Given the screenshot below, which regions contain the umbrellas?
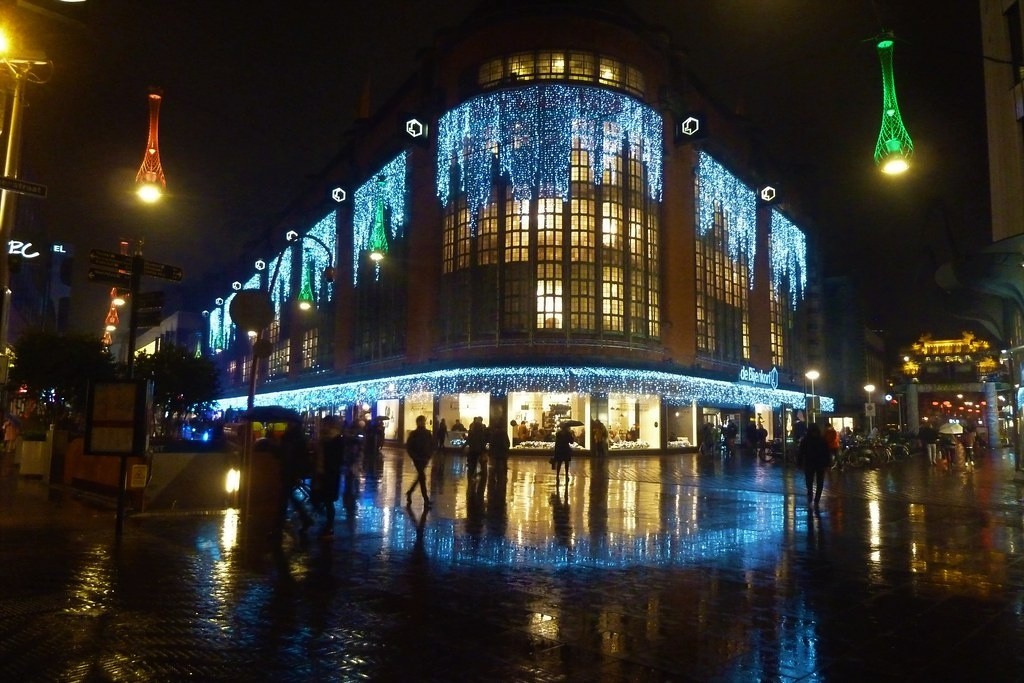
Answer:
[556,419,584,428]
[6,413,21,426]
[374,415,390,421]
[939,423,964,434]
[236,405,304,438]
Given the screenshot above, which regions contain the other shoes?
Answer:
[320,528,334,536]
[965,462,969,467]
[555,477,560,481]
[930,460,937,465]
[298,518,315,533]
[807,495,820,505]
[406,491,414,504]
[971,461,974,465]
[424,501,435,507]
[566,477,570,481]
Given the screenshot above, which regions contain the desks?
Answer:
[447,431,467,440]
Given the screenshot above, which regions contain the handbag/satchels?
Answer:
[549,459,557,470]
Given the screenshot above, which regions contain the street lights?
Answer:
[0,27,51,288]
[885,393,894,402]
[932,393,988,412]
[805,371,820,423]
[121,181,167,369]
[104,297,127,332]
[864,385,875,433]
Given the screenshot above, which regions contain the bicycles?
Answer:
[759,435,912,466]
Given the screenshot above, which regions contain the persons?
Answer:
[555,424,574,485]
[405,416,433,507]
[944,433,955,467]
[176,402,885,473]
[310,420,345,535]
[257,420,316,540]
[797,423,829,503]
[961,427,975,466]
[925,421,937,465]
[0,415,20,451]
